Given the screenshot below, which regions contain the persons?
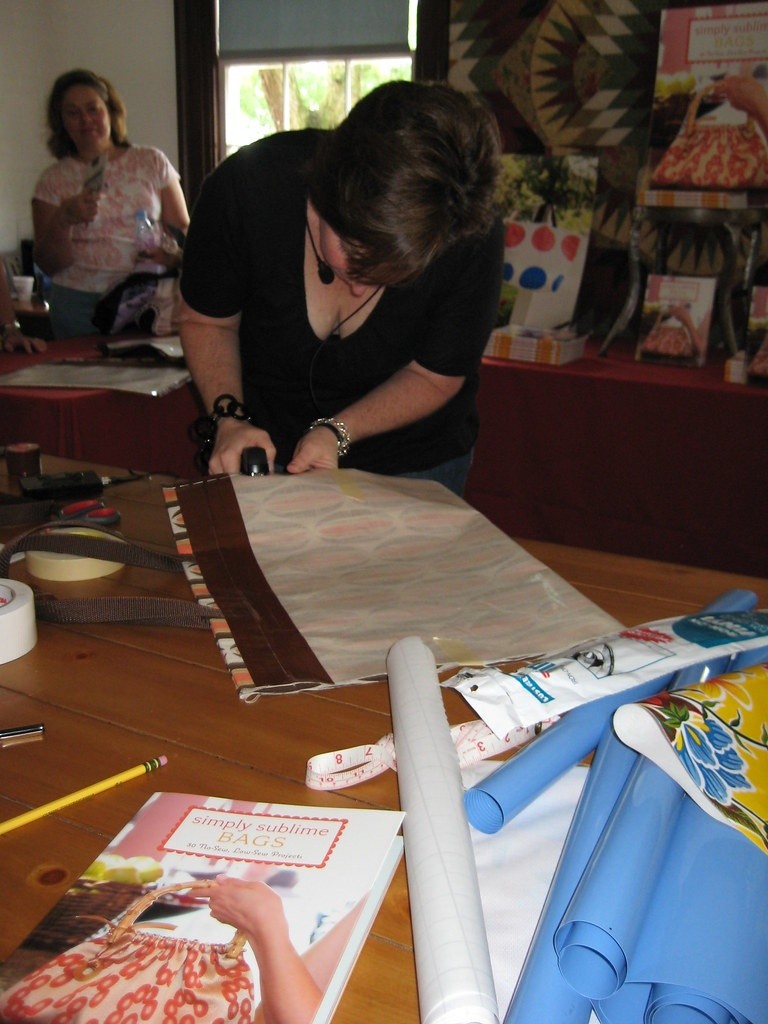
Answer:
[189,875,368,1024]
[32,70,191,336]
[726,76,768,124]
[0,260,47,355]
[182,80,502,498]
[665,307,707,354]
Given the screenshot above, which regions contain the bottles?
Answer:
[135,209,157,276]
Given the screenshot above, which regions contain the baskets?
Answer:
[23,877,157,950]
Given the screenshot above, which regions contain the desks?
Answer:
[0,341,768,579]
[0,453,768,1024]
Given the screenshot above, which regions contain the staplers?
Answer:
[241,446,269,477]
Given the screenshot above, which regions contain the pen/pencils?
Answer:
[0,723,45,740]
[63,357,123,365]
[0,754,168,835]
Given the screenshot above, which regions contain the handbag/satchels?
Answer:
[642,310,691,357]
[502,199,590,333]
[654,79,768,189]
[91,226,187,335]
[0,879,255,1024]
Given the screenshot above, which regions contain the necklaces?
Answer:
[330,283,383,333]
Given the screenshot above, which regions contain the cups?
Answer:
[12,276,34,303]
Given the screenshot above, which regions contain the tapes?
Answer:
[0,578,38,664]
[25,527,128,582]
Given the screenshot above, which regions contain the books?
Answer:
[645,5,768,186]
[745,286,768,377]
[0,792,406,1024]
[636,274,717,367]
[639,189,748,208]
[484,325,588,365]
[723,350,748,385]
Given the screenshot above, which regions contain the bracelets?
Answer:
[306,418,351,457]
[3,322,20,333]
[195,394,252,442]
